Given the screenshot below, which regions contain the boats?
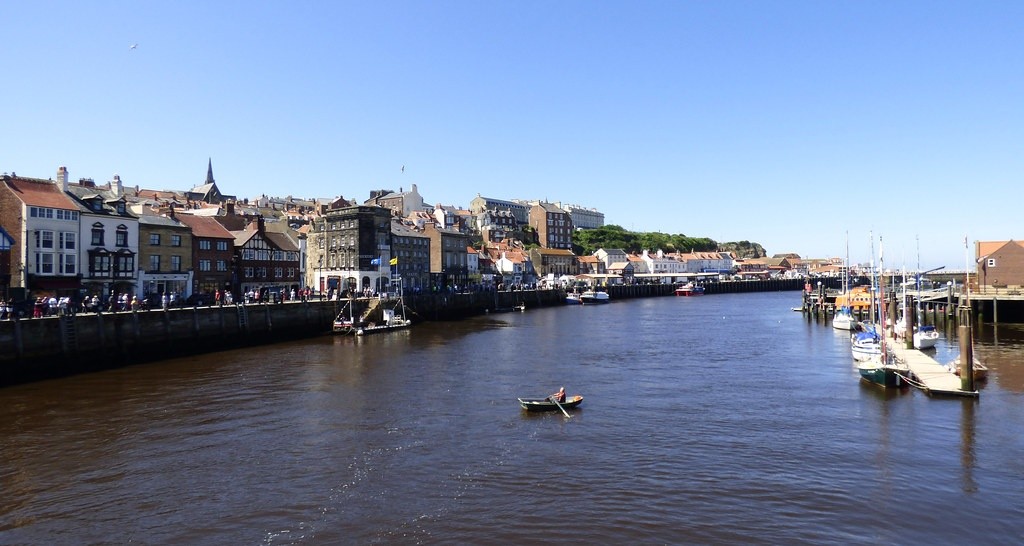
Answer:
[675,282,705,297]
[333,289,365,333]
[833,227,989,383]
[566,289,582,304]
[355,295,411,336]
[517,395,584,412]
[580,286,609,305]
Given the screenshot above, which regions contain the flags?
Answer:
[389,258,397,265]
[371,259,380,265]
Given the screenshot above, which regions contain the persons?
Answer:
[0,293,148,321]
[282,286,374,302]
[554,387,566,403]
[377,282,546,299]
[214,289,221,306]
[225,290,268,305]
[162,292,168,308]
[169,291,178,309]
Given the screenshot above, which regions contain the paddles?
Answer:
[552,396,570,419]
